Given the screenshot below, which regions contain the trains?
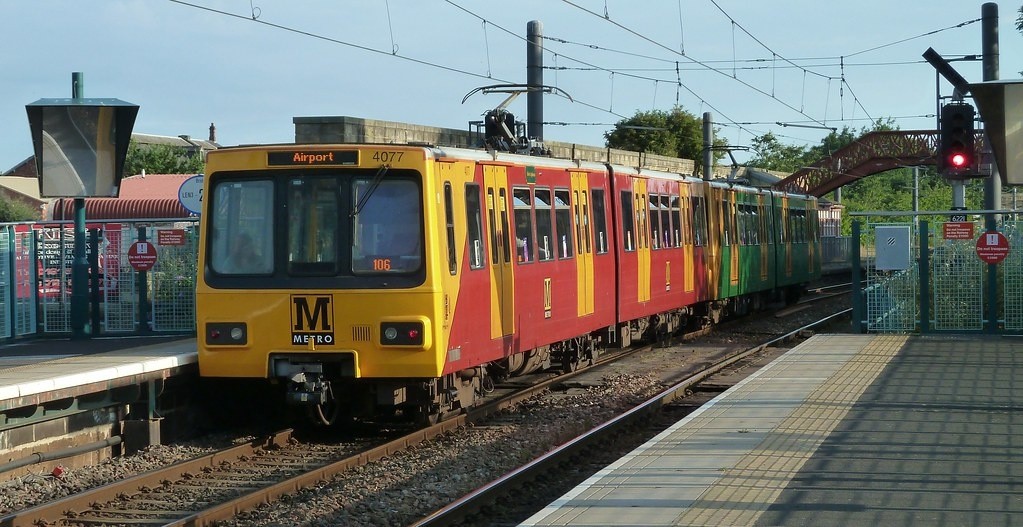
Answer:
[195,141,823,428]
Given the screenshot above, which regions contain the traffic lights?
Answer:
[940,103,974,176]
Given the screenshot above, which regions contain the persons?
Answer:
[222,234,262,273]
